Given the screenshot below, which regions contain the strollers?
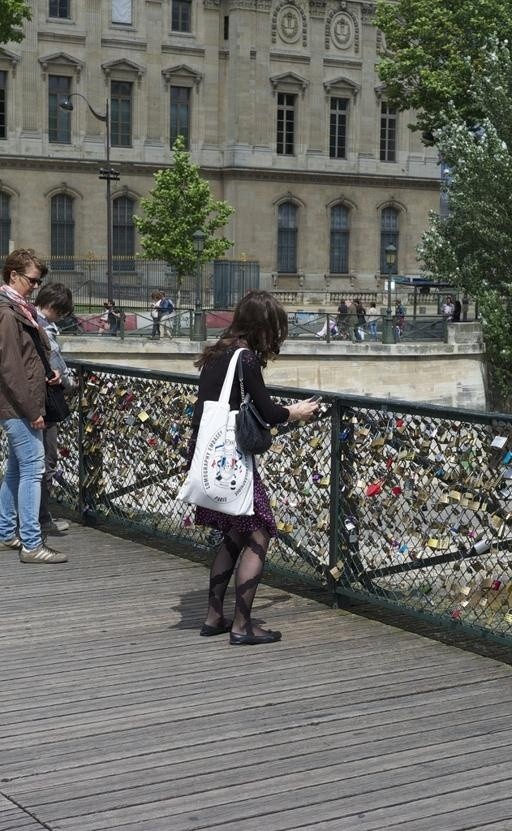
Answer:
[313,316,350,342]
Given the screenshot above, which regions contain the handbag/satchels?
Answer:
[46,372,69,422]
[175,401,255,516]
[236,392,271,455]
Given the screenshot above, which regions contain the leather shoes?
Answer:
[230,631,281,644]
[200,620,233,636]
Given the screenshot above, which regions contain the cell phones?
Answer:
[308,394,323,403]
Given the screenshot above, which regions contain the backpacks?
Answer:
[168,303,173,313]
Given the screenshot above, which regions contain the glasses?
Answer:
[18,272,42,286]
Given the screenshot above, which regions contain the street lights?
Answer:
[188,225,211,343]
[380,241,401,345]
[60,92,121,323]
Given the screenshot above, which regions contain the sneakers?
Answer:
[0,537,22,550]
[41,522,69,531]
[20,543,67,563]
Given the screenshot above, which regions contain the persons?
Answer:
[107,299,121,336]
[149,291,161,338]
[98,302,111,337]
[441,296,461,344]
[0,248,67,563]
[156,290,173,340]
[187,291,317,646]
[336,296,406,341]
[33,281,75,533]
